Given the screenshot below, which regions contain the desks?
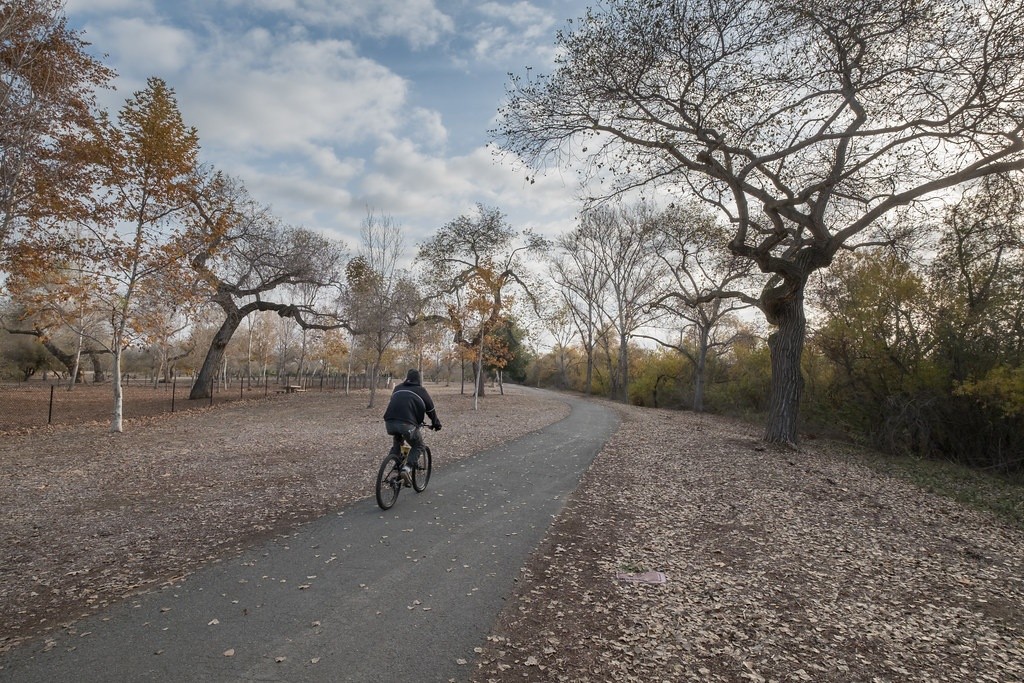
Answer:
[283,386,301,394]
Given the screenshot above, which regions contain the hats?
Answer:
[407,369,420,382]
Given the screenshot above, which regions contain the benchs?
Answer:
[273,391,285,392]
[295,390,307,392]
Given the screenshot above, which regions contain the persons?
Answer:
[383,369,442,473]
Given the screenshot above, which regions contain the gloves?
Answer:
[431,420,442,432]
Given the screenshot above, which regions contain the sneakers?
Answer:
[401,468,413,489]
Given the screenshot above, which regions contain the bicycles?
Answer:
[376,421,432,511]
